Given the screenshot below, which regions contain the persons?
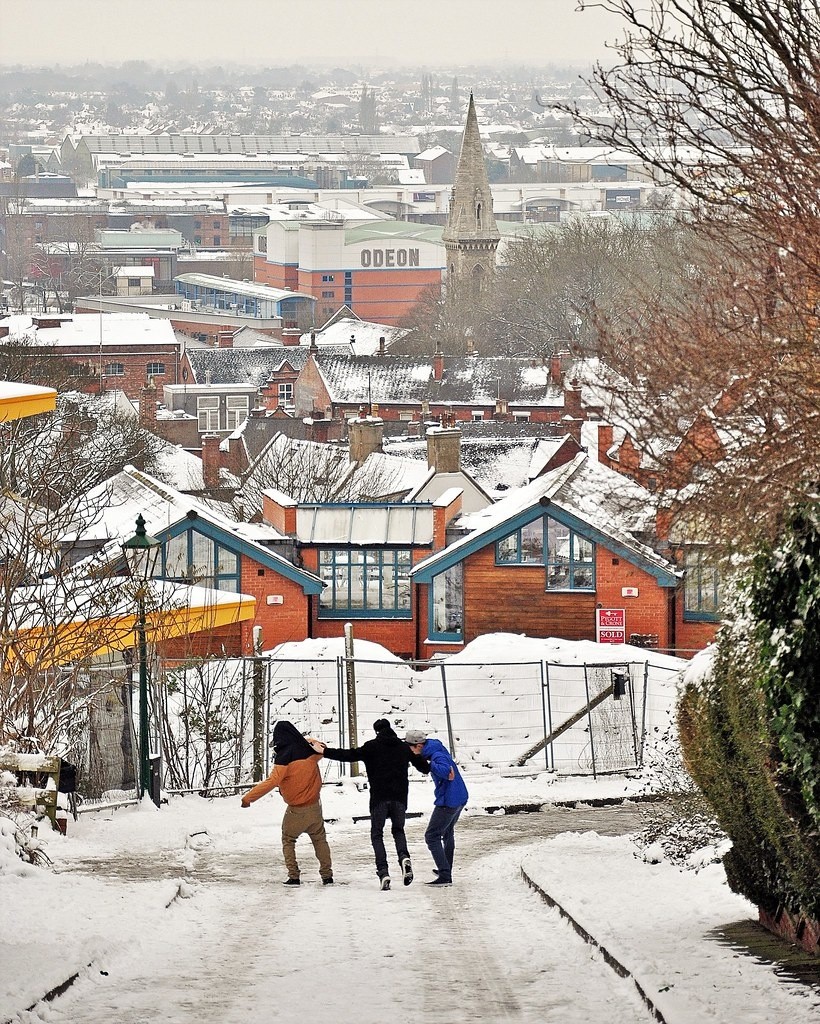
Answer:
[241,721,334,886]
[407,729,469,887]
[308,719,431,890]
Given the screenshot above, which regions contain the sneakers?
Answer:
[379,877,391,891]
[424,876,453,887]
[282,878,300,887]
[402,858,413,886]
[323,877,333,885]
[432,869,441,875]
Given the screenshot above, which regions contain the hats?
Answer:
[401,729,425,743]
